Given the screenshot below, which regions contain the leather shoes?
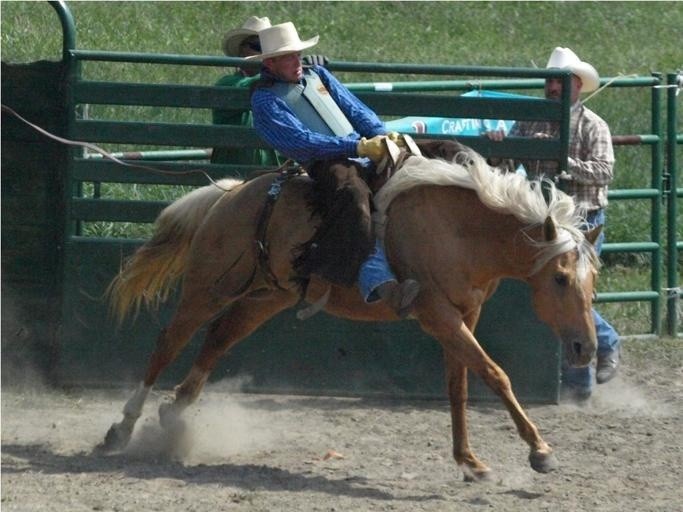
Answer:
[596,345,623,386]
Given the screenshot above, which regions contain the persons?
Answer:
[486,45,621,399]
[244,20,488,321]
[210,15,330,166]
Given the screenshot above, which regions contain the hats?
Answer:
[222,15,273,58]
[242,21,320,62]
[529,46,601,96]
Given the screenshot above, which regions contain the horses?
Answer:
[97,143,604,483]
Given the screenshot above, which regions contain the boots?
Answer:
[374,278,420,319]
